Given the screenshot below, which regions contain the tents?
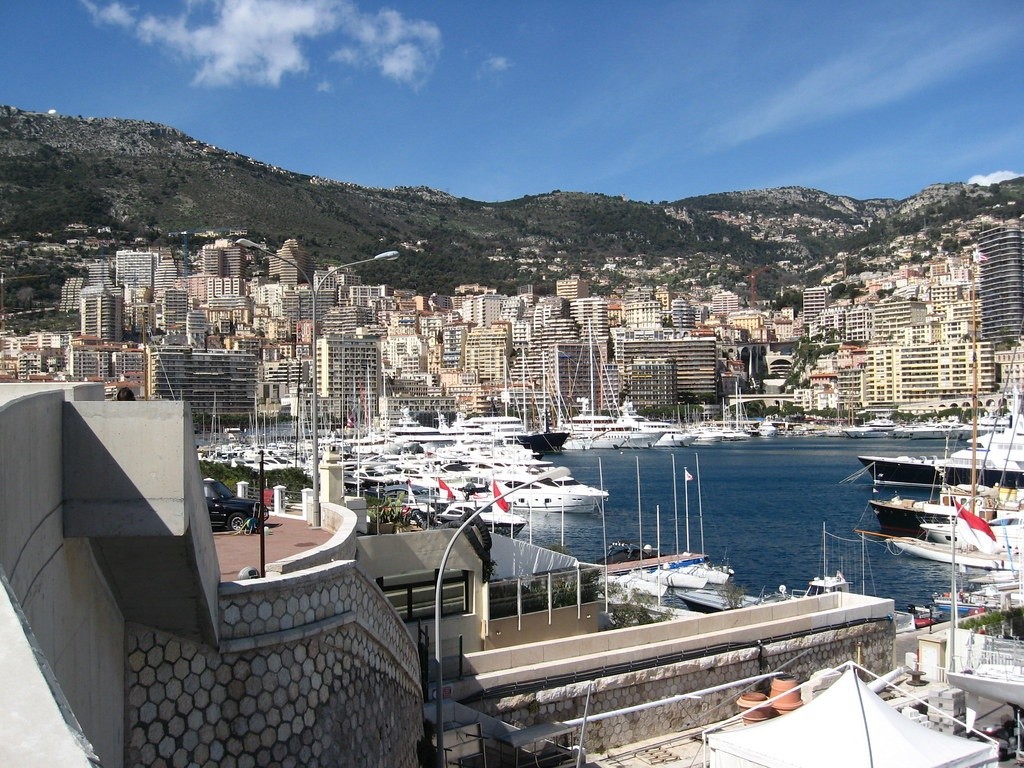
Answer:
[708,668,998,768]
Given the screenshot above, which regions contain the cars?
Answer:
[202,478,269,532]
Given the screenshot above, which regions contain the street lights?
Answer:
[235,237,399,526]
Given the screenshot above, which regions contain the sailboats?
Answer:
[197,318,1024,711]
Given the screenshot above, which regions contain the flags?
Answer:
[492,482,510,517]
[437,477,456,499]
[686,471,693,480]
[953,497,1004,555]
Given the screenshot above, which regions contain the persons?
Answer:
[117,387,135,400]
